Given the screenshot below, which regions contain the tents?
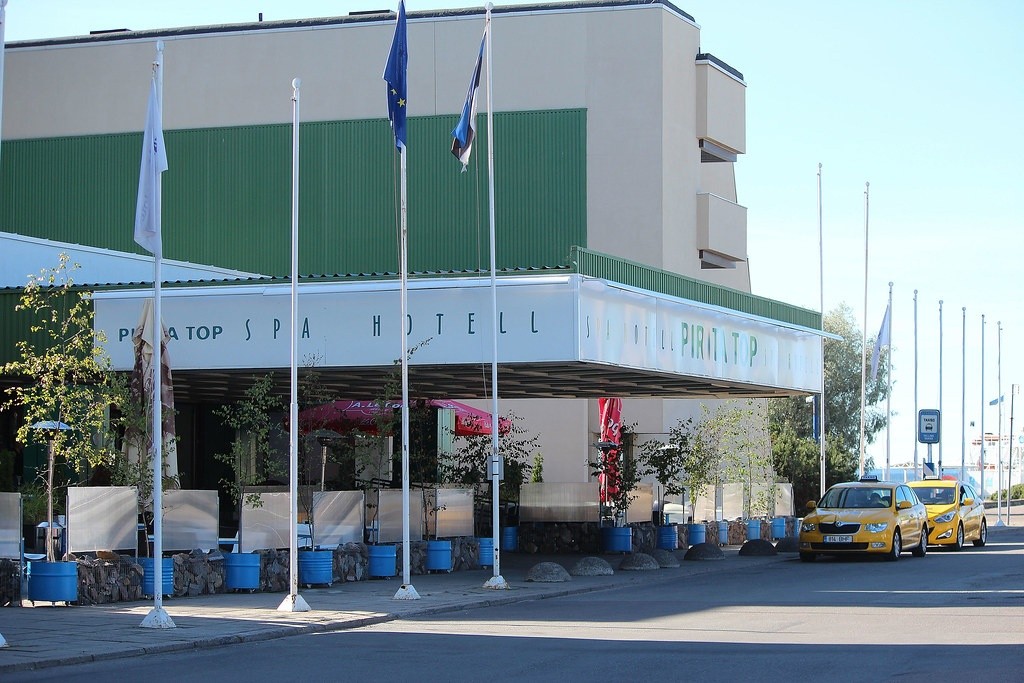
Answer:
[297,396,513,491]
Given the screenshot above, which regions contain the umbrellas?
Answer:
[597,397,623,509]
[116,296,181,516]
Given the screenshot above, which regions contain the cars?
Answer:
[903,474,989,552]
[796,474,930,560]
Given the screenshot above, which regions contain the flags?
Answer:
[989,395,1004,405]
[871,302,889,384]
[382,0,408,154]
[449,28,488,173]
[132,75,169,258]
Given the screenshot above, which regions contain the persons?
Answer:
[869,492,889,508]
